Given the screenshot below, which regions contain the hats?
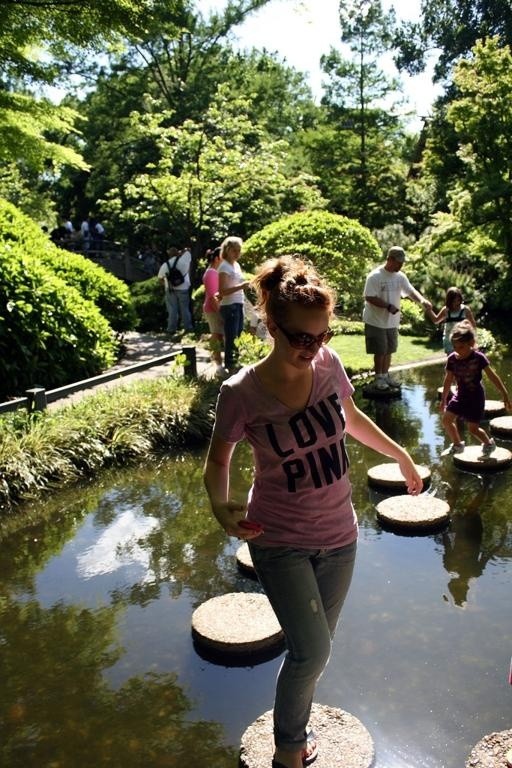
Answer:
[388,246,405,263]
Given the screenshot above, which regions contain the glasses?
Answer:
[269,313,335,350]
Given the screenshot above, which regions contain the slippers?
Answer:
[302,729,318,766]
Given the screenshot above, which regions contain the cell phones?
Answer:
[238,520,261,532]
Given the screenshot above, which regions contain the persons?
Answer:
[426,287,477,389]
[203,249,424,768]
[360,244,434,391]
[42,216,107,257]
[158,246,196,336]
[217,236,250,376]
[202,246,230,379]
[438,319,511,461]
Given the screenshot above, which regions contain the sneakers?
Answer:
[215,368,231,380]
[372,377,389,391]
[477,437,496,461]
[384,373,402,387]
[440,440,465,458]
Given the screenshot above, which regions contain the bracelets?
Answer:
[388,304,390,310]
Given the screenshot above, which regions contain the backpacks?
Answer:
[165,254,189,295]
